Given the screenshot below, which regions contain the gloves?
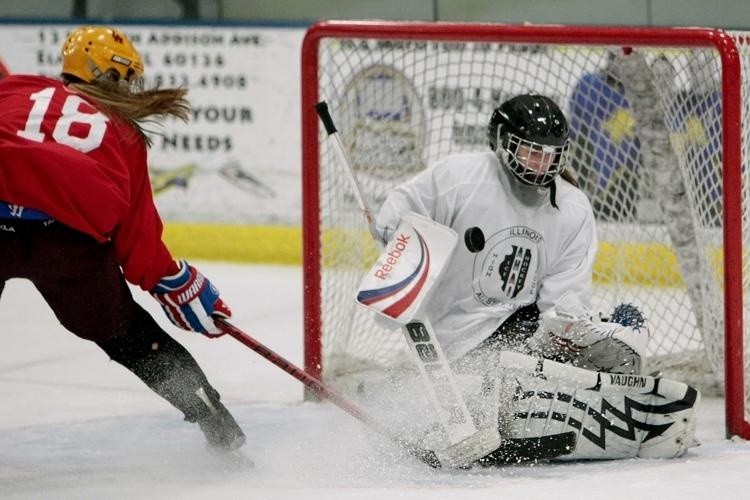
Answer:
[148,259,231,338]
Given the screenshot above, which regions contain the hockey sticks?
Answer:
[215,316,502,470]
[314,100,576,468]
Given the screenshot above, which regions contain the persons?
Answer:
[353,92,701,462]
[0,25,247,453]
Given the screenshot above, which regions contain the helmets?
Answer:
[63,27,143,87]
[488,95,570,208]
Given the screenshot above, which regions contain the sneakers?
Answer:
[198,400,246,449]
